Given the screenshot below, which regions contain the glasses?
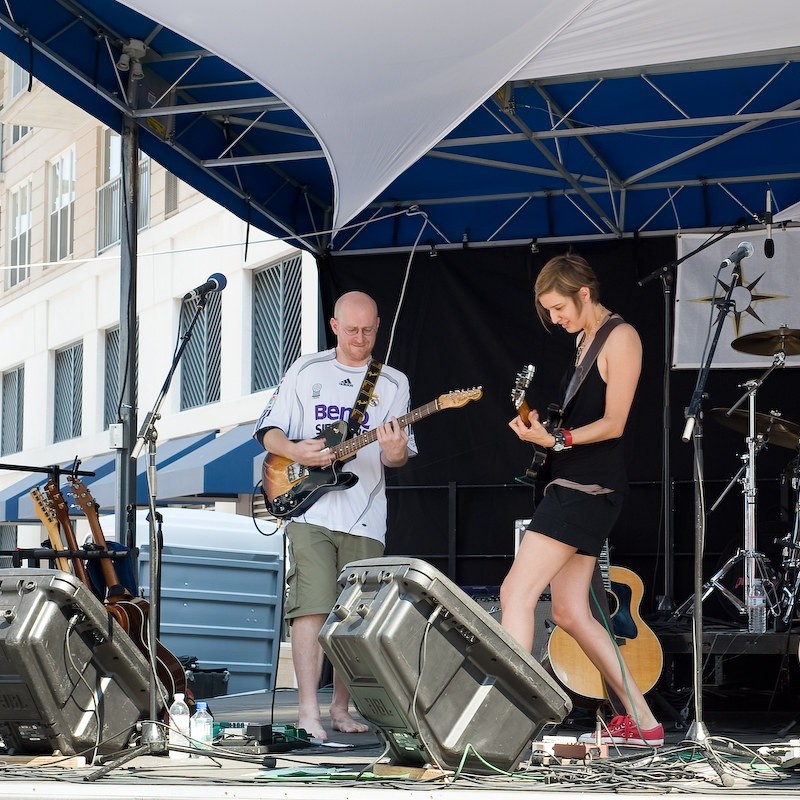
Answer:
[343,327,376,336]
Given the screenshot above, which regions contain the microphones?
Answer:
[719,241,754,268]
[183,272,227,300]
[764,191,774,258]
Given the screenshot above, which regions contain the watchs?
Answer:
[550,432,565,452]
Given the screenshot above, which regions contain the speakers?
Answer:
[318,557,573,777]
[0,568,169,760]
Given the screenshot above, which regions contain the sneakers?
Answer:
[578,715,664,748]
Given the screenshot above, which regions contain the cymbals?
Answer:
[731,327,800,356]
[711,408,800,450]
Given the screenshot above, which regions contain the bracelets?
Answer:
[561,429,572,450]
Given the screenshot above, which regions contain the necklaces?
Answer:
[576,306,605,364]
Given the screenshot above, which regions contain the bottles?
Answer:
[169,693,190,760]
[748,578,766,634]
[190,702,213,758]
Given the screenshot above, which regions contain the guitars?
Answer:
[29,475,188,701]
[262,385,486,520]
[547,536,664,710]
[510,365,563,504]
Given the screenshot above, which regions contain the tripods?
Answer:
[667,356,799,632]
[81,296,277,782]
[596,263,782,787]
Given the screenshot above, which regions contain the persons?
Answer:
[498,254,665,749]
[251,290,420,740]
